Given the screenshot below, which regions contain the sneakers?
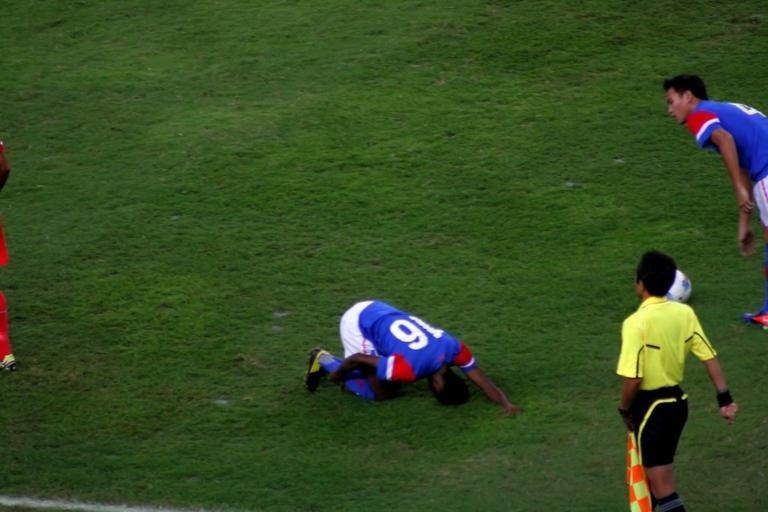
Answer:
[742,309,768,328]
[305,347,331,393]
[1,352,18,372]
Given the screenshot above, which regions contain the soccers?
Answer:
[665,271,692,303]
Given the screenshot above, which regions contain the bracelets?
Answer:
[715,386,733,407]
[617,405,628,417]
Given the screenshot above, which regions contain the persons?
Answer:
[665,75,768,329]
[304,301,525,416]
[617,250,738,512]
[2,138,18,374]
